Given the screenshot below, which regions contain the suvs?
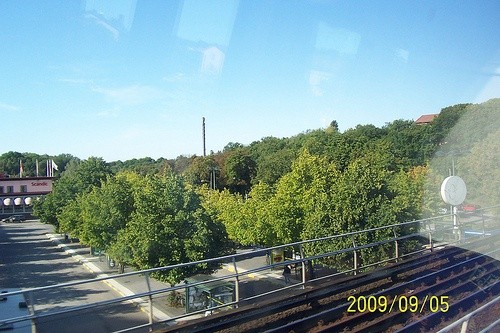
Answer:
[2,215,25,224]
